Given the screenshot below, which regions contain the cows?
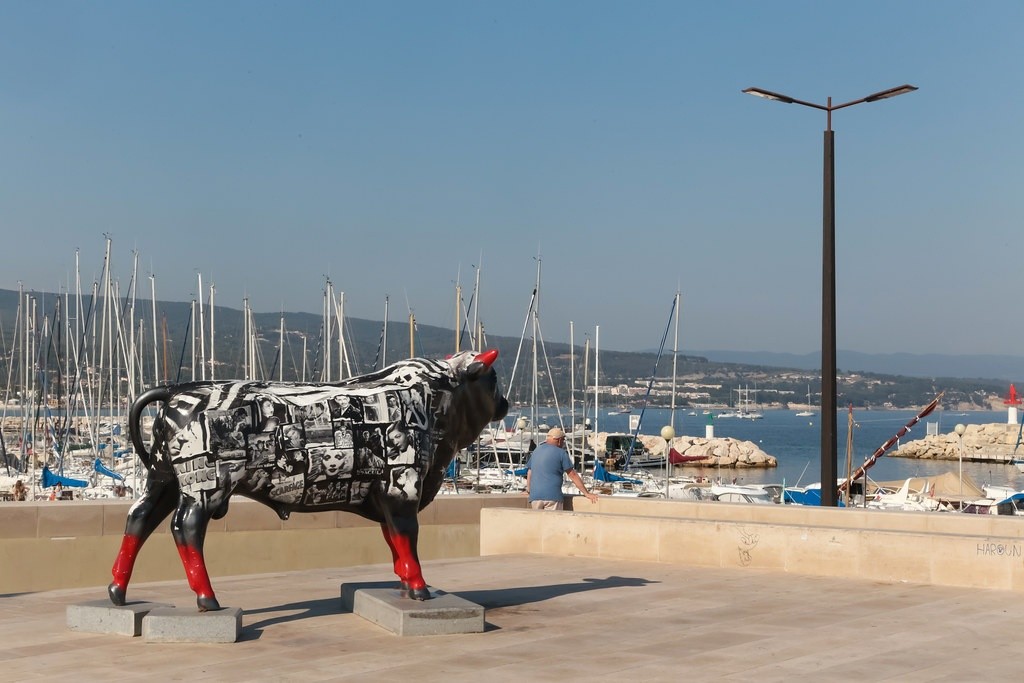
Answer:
[104,348,511,612]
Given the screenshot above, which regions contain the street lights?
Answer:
[741,84,920,507]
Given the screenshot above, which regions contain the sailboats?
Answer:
[0,230,1024,513]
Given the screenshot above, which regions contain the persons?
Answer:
[526,428,598,510]
[14,480,26,501]
[55,481,63,497]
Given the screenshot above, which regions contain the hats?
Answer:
[546,428,567,439]
[255,439,264,444]
